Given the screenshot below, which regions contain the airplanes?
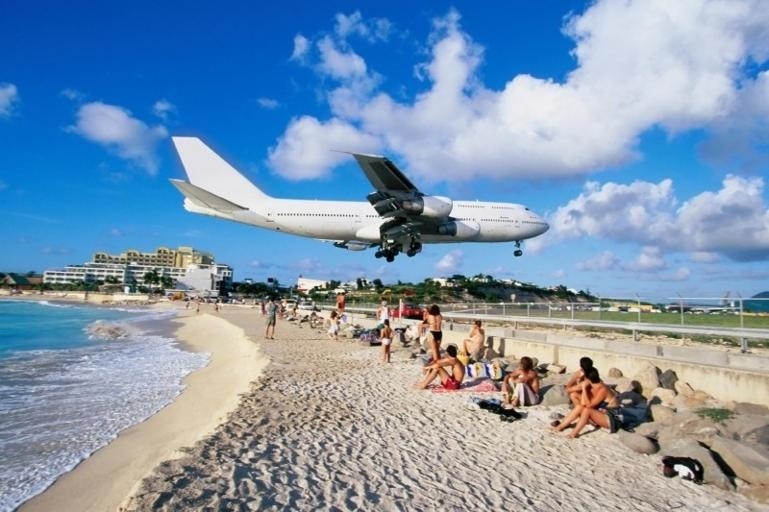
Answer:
[168,136,549,264]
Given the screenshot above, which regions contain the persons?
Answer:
[261,291,346,340]
[412,304,623,440]
[340,299,394,363]
[187,302,219,313]
